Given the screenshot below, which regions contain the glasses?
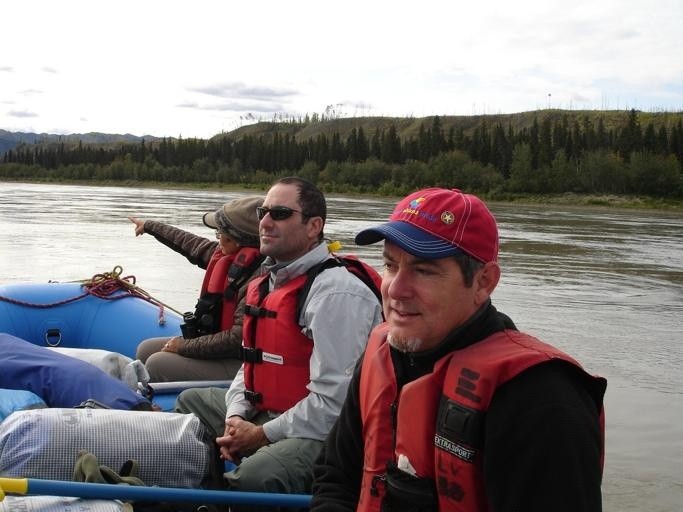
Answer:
[256,207,303,221]
[215,229,225,240]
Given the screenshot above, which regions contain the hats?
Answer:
[202,197,265,248]
[354,188,499,265]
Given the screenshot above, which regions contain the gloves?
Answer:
[70,450,147,487]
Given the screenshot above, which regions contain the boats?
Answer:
[0,265,236,473]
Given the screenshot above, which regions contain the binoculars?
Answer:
[179,312,194,339]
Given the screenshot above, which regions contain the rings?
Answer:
[163,343,169,348]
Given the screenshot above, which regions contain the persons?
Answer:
[171,174,388,511]
[123,194,269,387]
[306,183,607,511]
